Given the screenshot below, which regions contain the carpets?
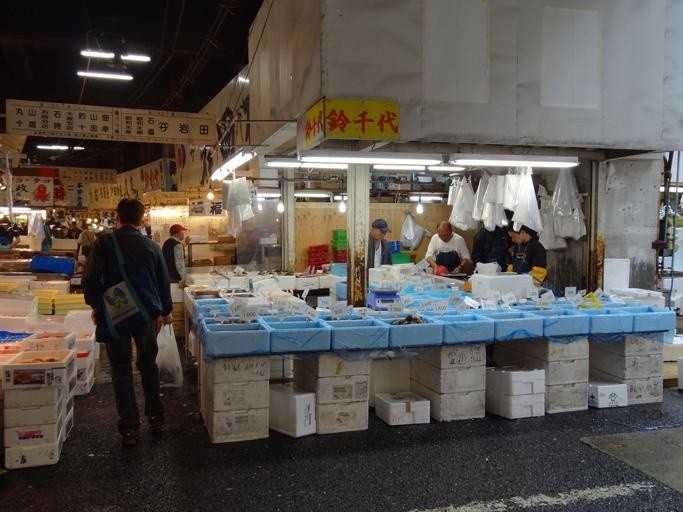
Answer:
[579,426,683,496]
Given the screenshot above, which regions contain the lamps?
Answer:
[257,192,282,198]
[211,151,258,181]
[37,145,85,151]
[265,161,349,169]
[80,50,115,58]
[120,54,151,62]
[427,165,467,172]
[301,149,444,166]
[416,204,424,215]
[373,164,427,172]
[294,193,331,198]
[77,71,133,81]
[277,203,284,213]
[208,191,214,200]
[338,201,347,212]
[450,154,582,169]
[406,195,443,202]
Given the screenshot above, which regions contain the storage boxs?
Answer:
[588,377,629,409]
[331,230,347,263]
[573,289,677,334]
[369,358,413,407]
[486,388,545,419]
[590,332,663,357]
[485,365,545,395]
[486,296,589,341]
[0,274,99,471]
[30,255,76,275]
[409,358,486,394]
[157,303,183,322]
[157,321,184,339]
[171,283,183,302]
[374,391,430,426]
[418,342,486,369]
[183,264,391,357]
[472,273,531,297]
[200,391,269,444]
[185,236,237,266]
[382,285,494,347]
[293,360,369,404]
[270,355,295,381]
[410,378,485,422]
[269,383,316,438]
[200,356,270,384]
[546,381,588,415]
[511,348,590,386]
[315,400,369,435]
[51,238,78,251]
[661,363,679,379]
[510,335,590,363]
[387,241,412,264]
[200,373,270,412]
[589,365,664,405]
[590,344,662,379]
[303,351,369,378]
[13,236,41,251]
[307,244,329,264]
[660,334,683,362]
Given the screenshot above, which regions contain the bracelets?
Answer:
[459,265,464,268]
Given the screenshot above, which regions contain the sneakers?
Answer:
[116,420,139,447]
[147,413,165,427]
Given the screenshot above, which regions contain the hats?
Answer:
[371,218,392,236]
[168,222,188,236]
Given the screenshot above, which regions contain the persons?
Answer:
[426,221,470,274]
[368,219,391,268]
[45,215,79,239]
[162,225,192,287]
[506,224,549,284]
[84,198,183,436]
[0,215,28,249]
[77,223,96,253]
